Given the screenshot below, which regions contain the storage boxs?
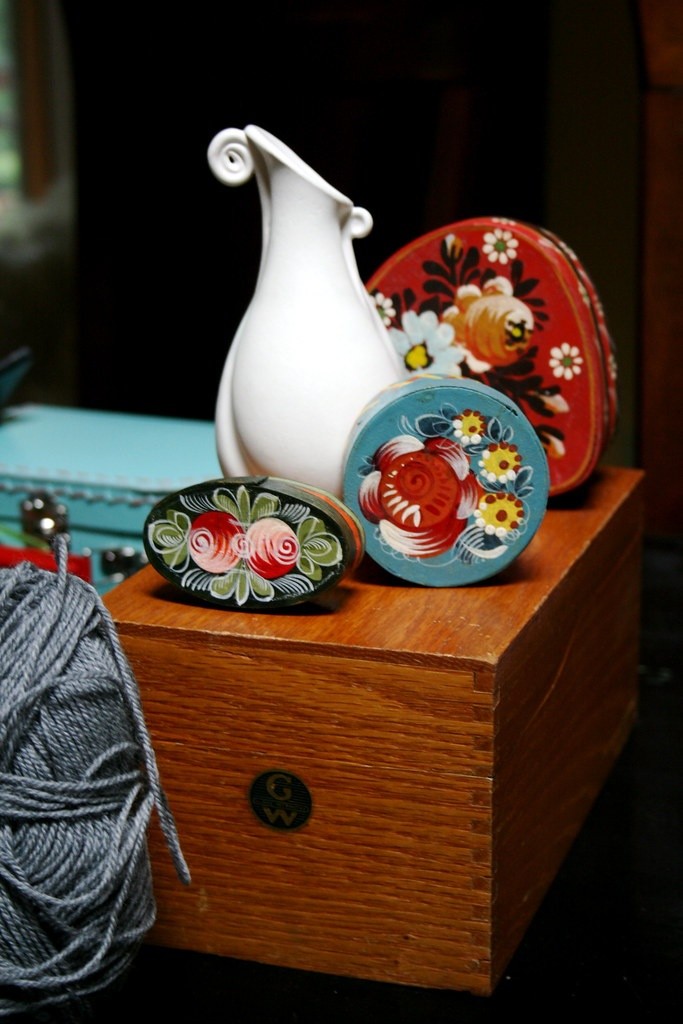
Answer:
[0,396,649,995]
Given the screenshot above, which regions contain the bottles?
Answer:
[207,123,408,498]
